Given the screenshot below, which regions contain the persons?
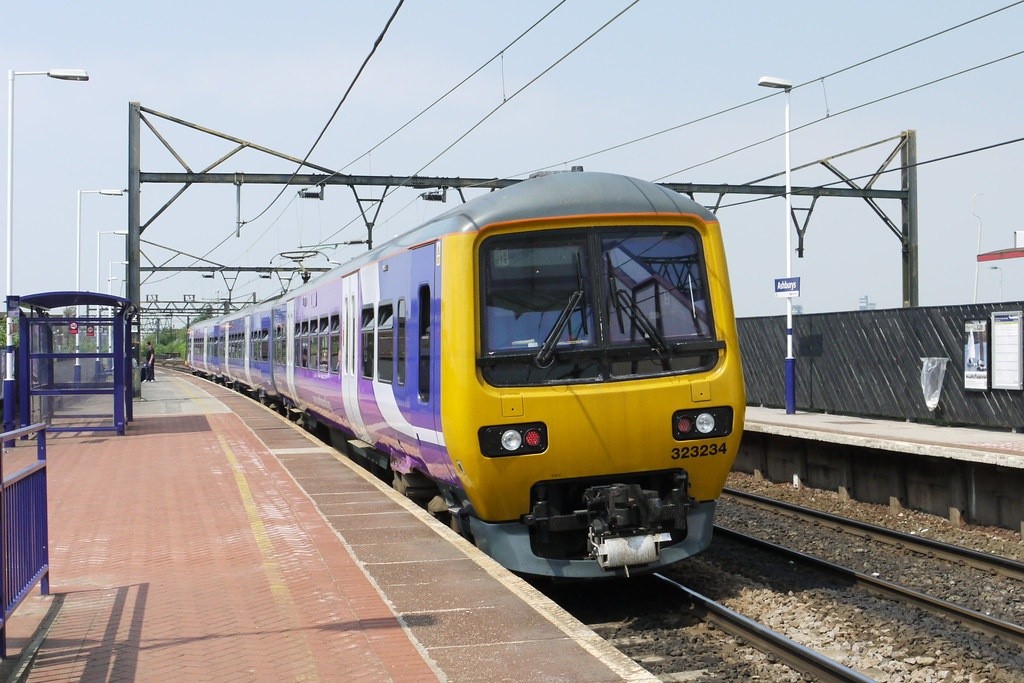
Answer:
[143,340,156,383]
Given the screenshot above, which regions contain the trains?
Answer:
[182,163,747,584]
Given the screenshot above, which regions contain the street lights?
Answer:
[94,230,129,380]
[3,66,90,448]
[72,189,123,384]
[108,260,130,372]
[758,75,799,415]
